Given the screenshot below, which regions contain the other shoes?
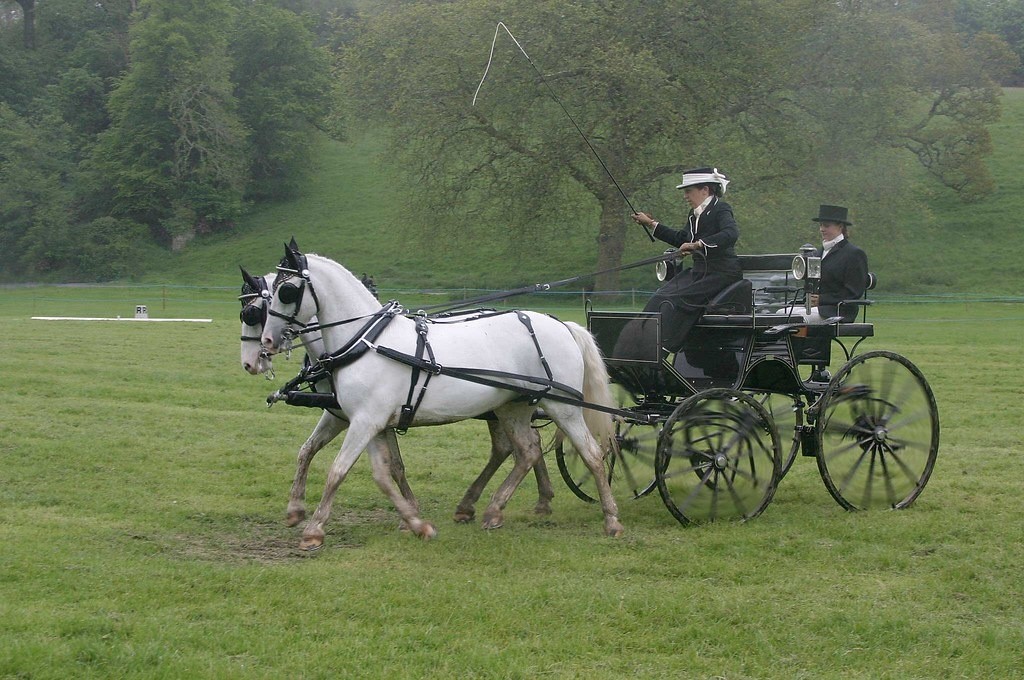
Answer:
[631,394,668,410]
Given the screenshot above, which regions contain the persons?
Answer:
[613,166,743,362]
[777,204,868,330]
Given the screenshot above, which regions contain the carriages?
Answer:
[236,243,941,553]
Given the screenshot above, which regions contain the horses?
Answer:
[236,235,627,551]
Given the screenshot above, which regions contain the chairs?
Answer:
[697,254,806,325]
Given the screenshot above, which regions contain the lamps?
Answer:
[791,254,822,282]
[656,260,674,282]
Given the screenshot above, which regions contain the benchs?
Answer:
[807,273,877,336]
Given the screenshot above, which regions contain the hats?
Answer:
[676,167,730,195]
[812,204,853,226]
